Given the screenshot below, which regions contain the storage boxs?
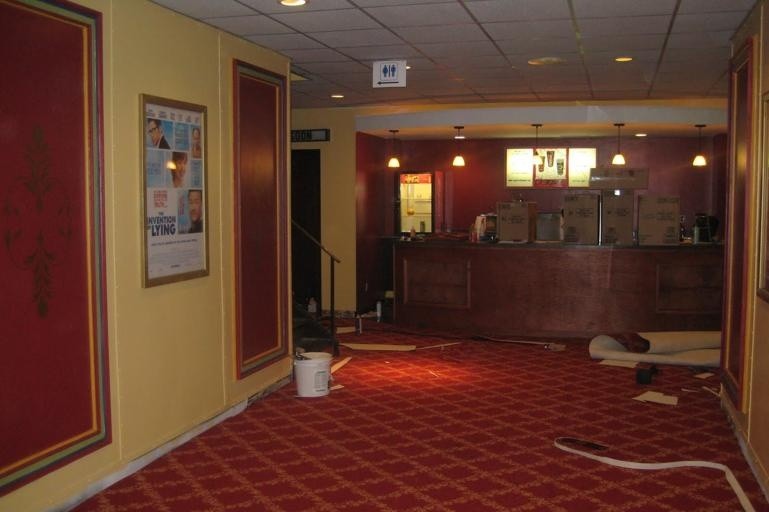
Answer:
[561,164,682,246]
[493,199,541,244]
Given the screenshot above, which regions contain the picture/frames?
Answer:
[137,91,210,289]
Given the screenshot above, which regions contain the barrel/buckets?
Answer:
[294,358,331,397]
[301,351,332,360]
[474,212,488,241]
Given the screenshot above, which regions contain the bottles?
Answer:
[410,226,416,241]
[420,222,425,232]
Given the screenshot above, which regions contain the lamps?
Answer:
[453,125,467,168]
[387,128,402,169]
[611,123,629,165]
[691,124,707,168]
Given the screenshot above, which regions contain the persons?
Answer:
[147,118,172,149]
[168,151,188,188]
[190,128,202,160]
[186,190,203,233]
